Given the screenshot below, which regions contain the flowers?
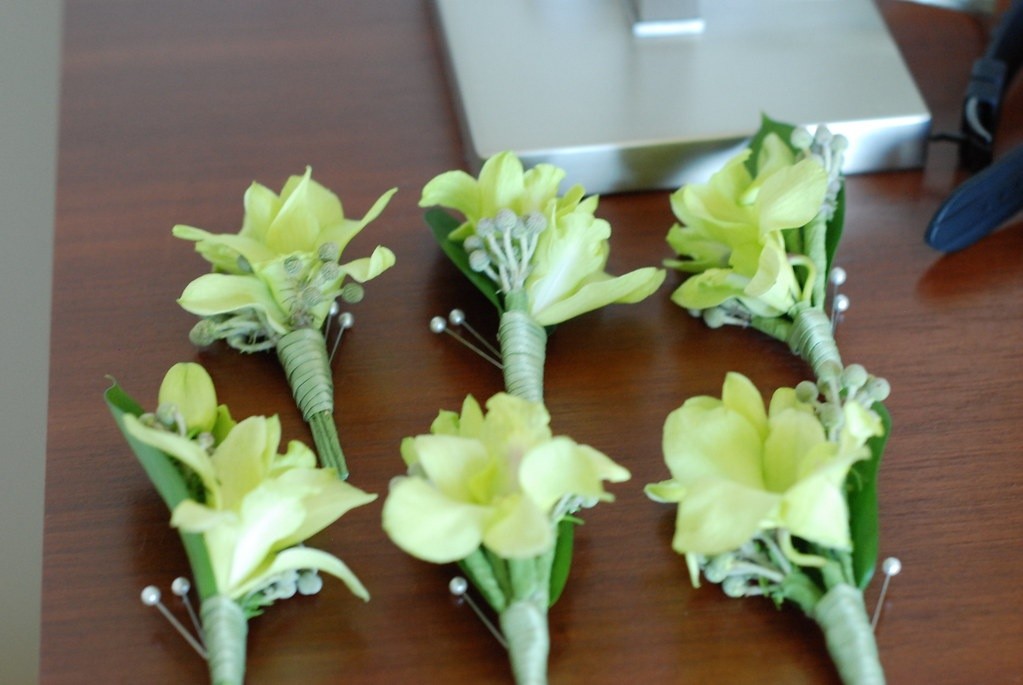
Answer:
[380,393,631,685]
[662,108,848,377]
[104,362,379,685]
[419,150,666,405]
[174,165,399,481]
[644,364,901,685]
